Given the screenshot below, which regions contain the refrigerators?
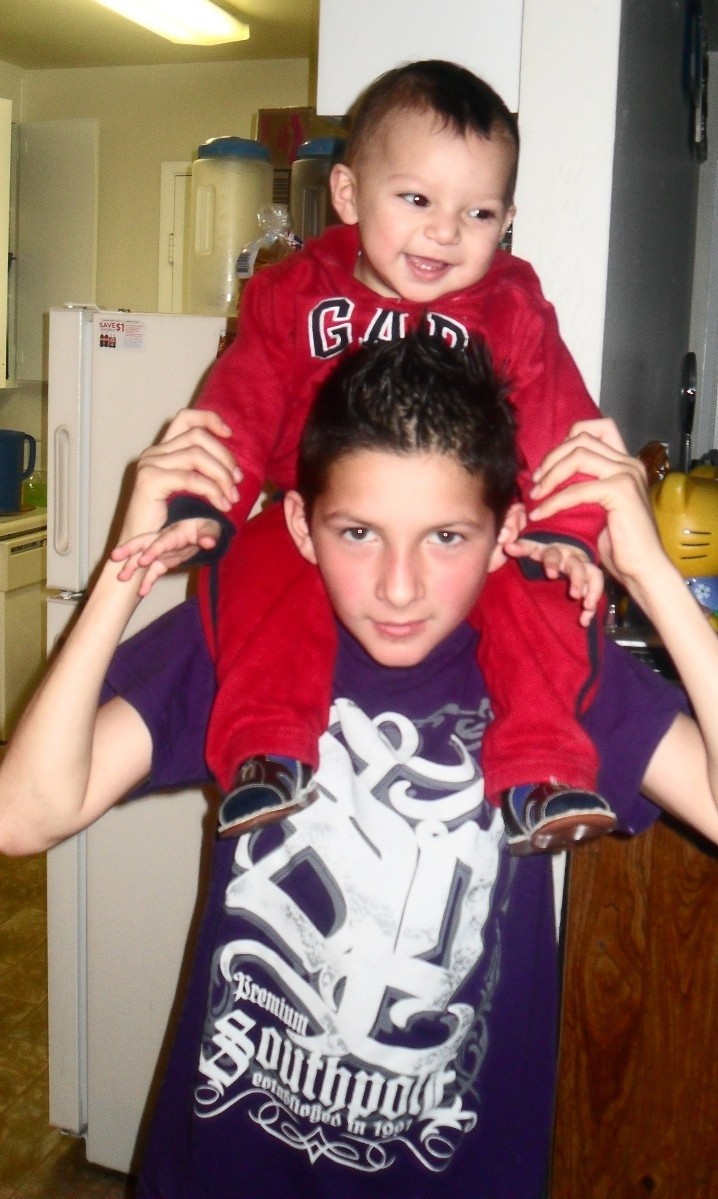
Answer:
[42,303,229,1171]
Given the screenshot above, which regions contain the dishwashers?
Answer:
[0,530,47,746]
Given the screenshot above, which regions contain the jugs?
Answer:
[0,428,38,514]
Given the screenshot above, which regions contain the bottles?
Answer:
[185,135,275,320]
[288,137,348,244]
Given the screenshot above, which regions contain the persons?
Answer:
[0,324,718,1198]
[111,60,617,856]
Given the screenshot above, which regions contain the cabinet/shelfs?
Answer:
[0,527,50,741]
[0,100,101,391]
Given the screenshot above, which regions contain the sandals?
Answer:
[496,779,617,854]
[218,755,316,838]
